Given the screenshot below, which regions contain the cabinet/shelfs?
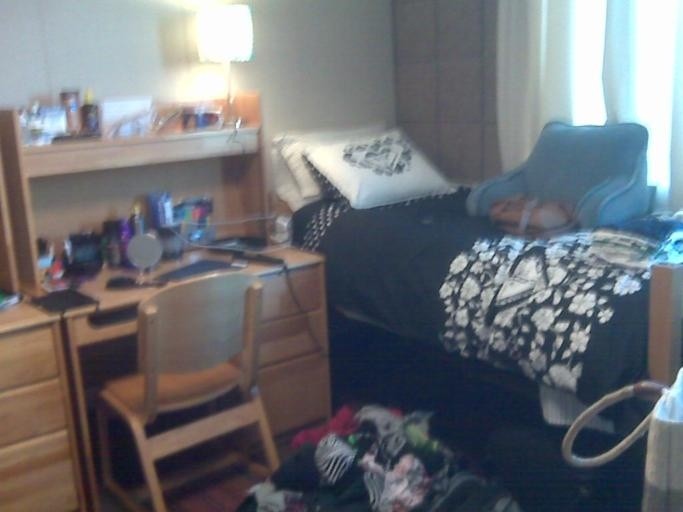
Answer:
[246,268,330,457]
[0,92,274,300]
[0,324,82,511]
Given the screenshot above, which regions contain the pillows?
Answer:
[280,127,459,210]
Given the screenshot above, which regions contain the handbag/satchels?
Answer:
[488,194,577,237]
[559,362,683,510]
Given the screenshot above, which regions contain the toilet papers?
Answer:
[100,96,153,131]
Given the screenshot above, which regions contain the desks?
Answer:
[38,240,325,512]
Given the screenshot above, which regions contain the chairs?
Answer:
[87,271,280,512]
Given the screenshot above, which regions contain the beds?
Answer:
[269,172,681,411]
